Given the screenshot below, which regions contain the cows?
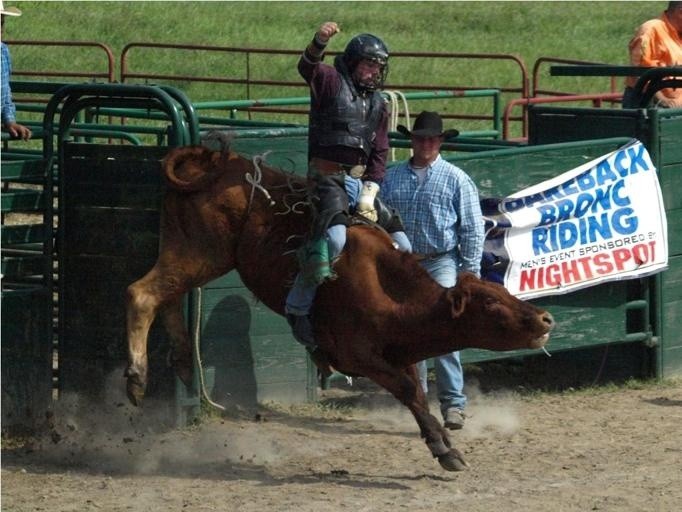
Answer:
[121,130,556,474]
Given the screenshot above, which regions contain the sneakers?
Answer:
[284,311,315,346]
[444,407,468,430]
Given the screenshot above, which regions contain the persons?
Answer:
[619,2,681,111]
[282,21,392,353]
[1,2,33,235]
[378,110,487,431]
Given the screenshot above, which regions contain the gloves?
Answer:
[355,184,378,223]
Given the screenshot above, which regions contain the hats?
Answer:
[397,110,459,142]
[0,0,23,18]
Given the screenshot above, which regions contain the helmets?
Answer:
[343,33,390,90]
